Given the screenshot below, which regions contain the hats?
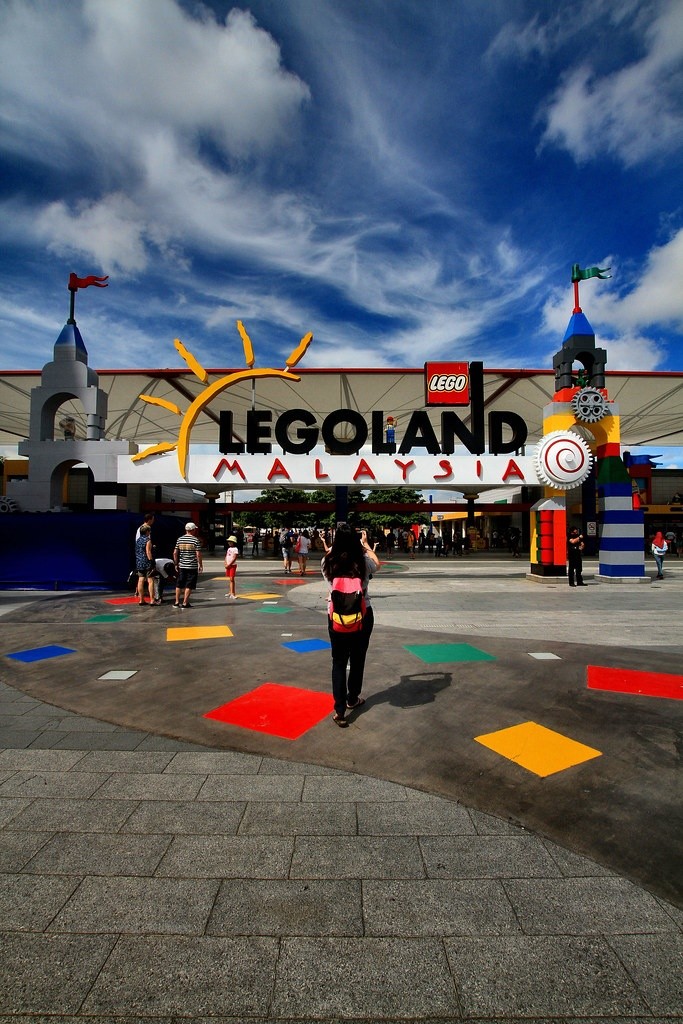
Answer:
[226,536,238,543]
[185,522,198,531]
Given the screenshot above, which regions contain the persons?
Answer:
[234,523,523,576]
[135,514,154,542]
[224,535,239,599]
[651,531,668,579]
[172,522,203,609]
[566,527,587,587]
[646,523,683,558]
[153,557,174,602]
[320,523,380,728]
[135,524,161,606]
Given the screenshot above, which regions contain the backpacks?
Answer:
[280,531,290,548]
[329,576,366,633]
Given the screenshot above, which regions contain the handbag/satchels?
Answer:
[295,535,301,552]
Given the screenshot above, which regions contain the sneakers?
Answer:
[172,603,179,609]
[181,604,192,610]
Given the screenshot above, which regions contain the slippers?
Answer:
[347,698,366,709]
[332,715,349,728]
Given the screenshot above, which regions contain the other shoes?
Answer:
[288,569,292,573]
[569,584,576,587]
[300,572,306,576]
[139,602,148,606]
[284,569,288,574]
[134,592,139,597]
[231,594,237,599]
[225,593,230,598]
[387,556,392,559]
[150,603,161,606]
[577,582,588,586]
[409,557,415,560]
